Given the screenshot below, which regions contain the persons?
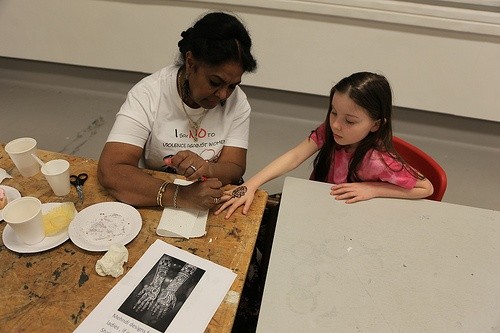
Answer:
[213,71,434,219]
[97,13,257,211]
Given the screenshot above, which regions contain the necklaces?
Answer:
[176,67,204,142]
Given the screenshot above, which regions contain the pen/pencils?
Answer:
[197,176,235,197]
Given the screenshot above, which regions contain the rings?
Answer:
[190,165,197,173]
[215,198,218,204]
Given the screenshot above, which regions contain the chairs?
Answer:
[390,137,448,201]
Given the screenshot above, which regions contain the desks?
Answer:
[254,176,500,333]
[0,144,267,333]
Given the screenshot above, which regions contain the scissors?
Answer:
[68,172,91,203]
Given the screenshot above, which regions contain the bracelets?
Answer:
[173,184,179,208]
[156,180,170,208]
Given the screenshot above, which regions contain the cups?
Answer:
[41,159,71,196]
[5,137,41,177]
[2,196,45,244]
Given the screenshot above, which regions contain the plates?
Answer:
[68,201,142,252]
[0,184,22,222]
[2,202,78,254]
